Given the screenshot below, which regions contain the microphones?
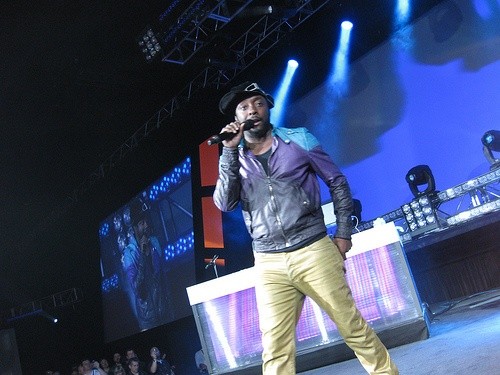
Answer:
[143,228,149,252]
[208,119,254,145]
[205,254,220,269]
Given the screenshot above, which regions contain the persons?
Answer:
[213,82,399,375]
[123,201,174,333]
[45,345,207,375]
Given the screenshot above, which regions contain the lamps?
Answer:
[400,165,441,201]
[481,129,500,171]
[400,193,441,239]
[135,27,171,67]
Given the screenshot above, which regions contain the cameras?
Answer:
[154,351,160,357]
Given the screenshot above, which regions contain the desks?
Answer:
[186,221,430,375]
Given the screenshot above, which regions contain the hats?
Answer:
[218,80,274,118]
[130,204,152,226]
